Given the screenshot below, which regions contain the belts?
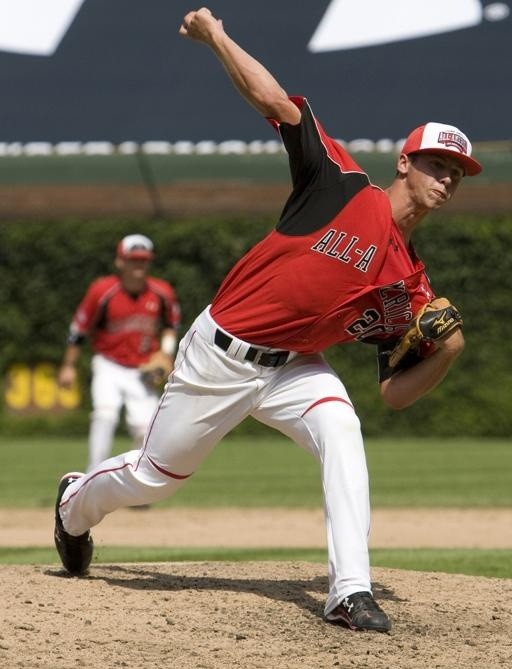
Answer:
[214,328,290,367]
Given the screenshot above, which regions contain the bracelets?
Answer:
[161,336,176,356]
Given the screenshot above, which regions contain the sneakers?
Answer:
[54,471,94,574]
[326,591,393,632]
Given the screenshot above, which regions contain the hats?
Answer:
[401,121,482,177]
[117,233,155,261]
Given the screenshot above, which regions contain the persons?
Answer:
[55,7,483,630]
[59,234,183,473]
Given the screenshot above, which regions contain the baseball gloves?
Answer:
[140,353,173,388]
[384,298,463,373]
